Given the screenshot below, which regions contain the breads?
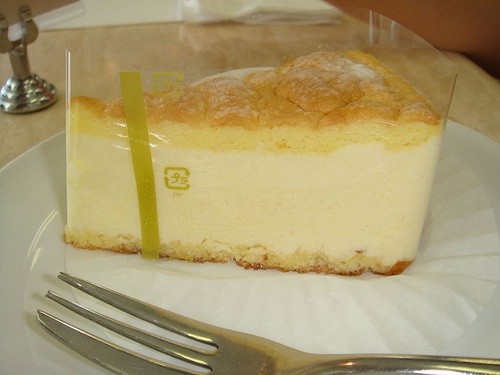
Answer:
[64,49,445,277]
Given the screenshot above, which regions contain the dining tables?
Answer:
[0,22,499,168]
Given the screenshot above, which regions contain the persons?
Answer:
[326,0,500,81]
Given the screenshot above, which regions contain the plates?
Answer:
[0,67,500,375]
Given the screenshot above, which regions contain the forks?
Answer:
[37,271,500,375]
